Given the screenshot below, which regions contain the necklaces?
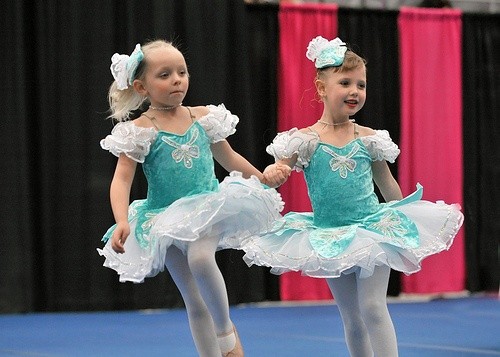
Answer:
[317,119,355,127]
[148,104,181,111]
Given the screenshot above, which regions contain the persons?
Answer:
[263,36,408,357]
[110,37,291,357]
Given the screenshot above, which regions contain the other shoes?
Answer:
[217,319,244,357]
